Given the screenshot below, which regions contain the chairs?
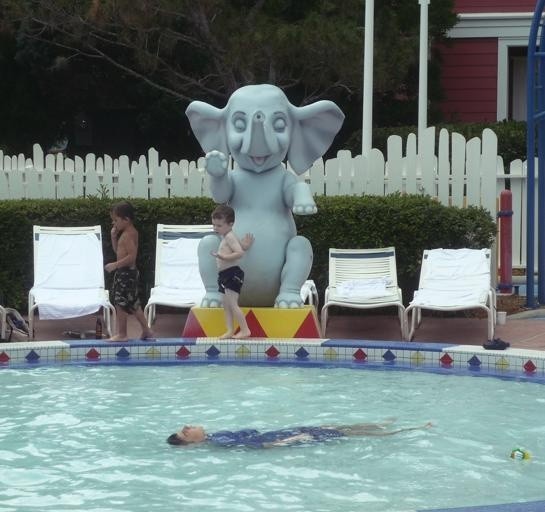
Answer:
[403,247,497,343]
[27,224,117,342]
[143,223,223,328]
[320,245,408,342]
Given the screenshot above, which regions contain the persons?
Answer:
[209,204,255,339]
[103,200,154,342]
[166,416,432,451]
